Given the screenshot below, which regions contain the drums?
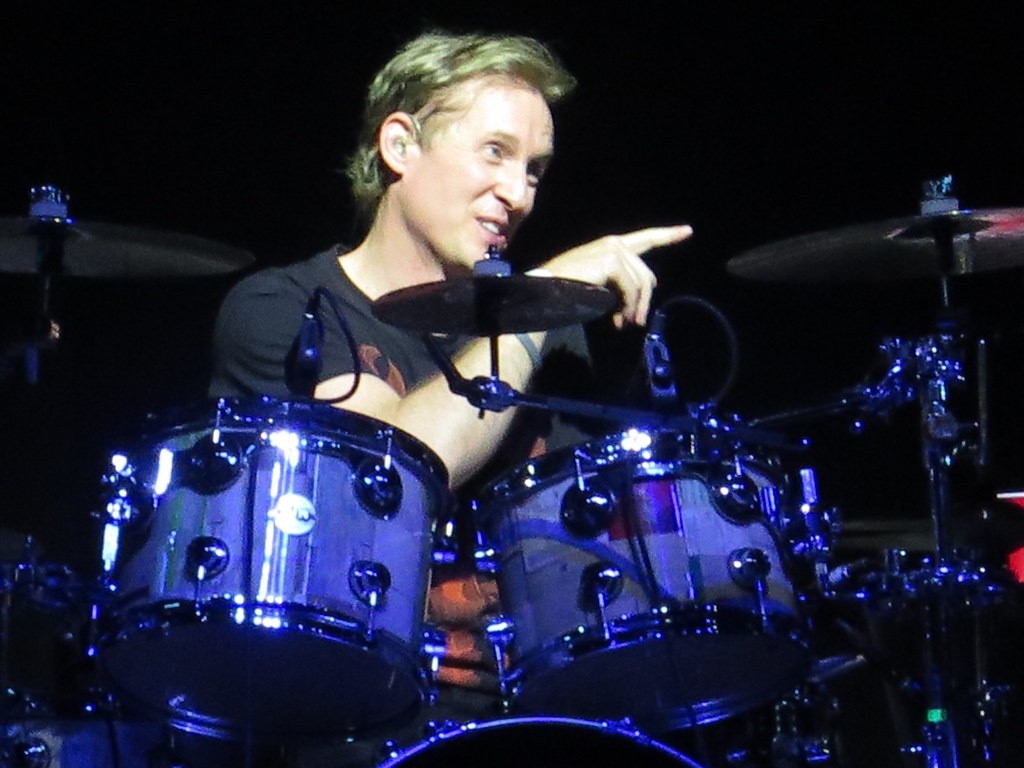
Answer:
[466,427,840,739]
[750,649,935,768]
[94,387,450,744]
[376,711,699,768]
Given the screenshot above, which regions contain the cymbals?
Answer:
[734,204,1024,293]
[2,211,243,282]
[368,275,615,338]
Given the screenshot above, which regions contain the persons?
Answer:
[214,30,692,690]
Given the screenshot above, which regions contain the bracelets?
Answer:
[514,330,543,368]
